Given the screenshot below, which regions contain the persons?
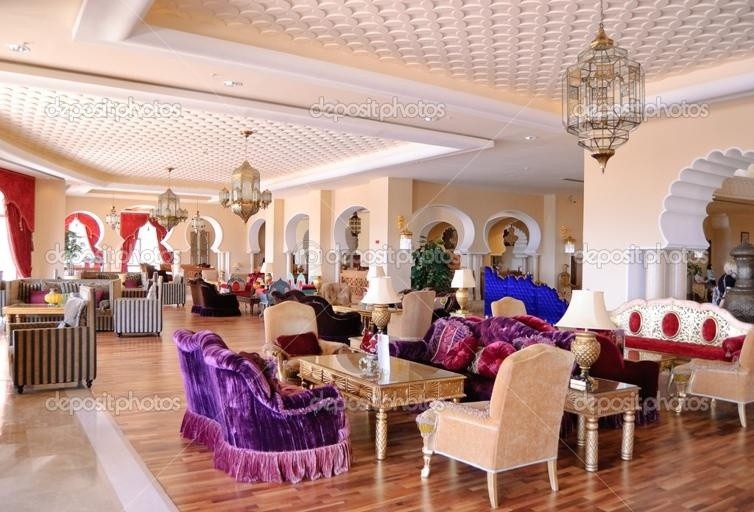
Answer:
[695,262,736,308]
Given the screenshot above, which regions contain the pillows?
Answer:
[146,282,156,299]
[63,297,87,326]
[20,282,108,307]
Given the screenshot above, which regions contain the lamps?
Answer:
[191,212,205,233]
[220,131,271,224]
[561,0,645,174]
[397,215,412,250]
[563,237,576,253]
[105,206,120,229]
[450,269,476,314]
[150,168,188,233]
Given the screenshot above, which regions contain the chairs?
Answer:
[140,272,185,306]
[140,263,168,282]
[113,276,163,338]
[7,287,96,393]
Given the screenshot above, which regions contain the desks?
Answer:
[2,303,64,323]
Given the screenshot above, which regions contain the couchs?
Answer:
[80,272,148,298]
[6,279,121,331]
